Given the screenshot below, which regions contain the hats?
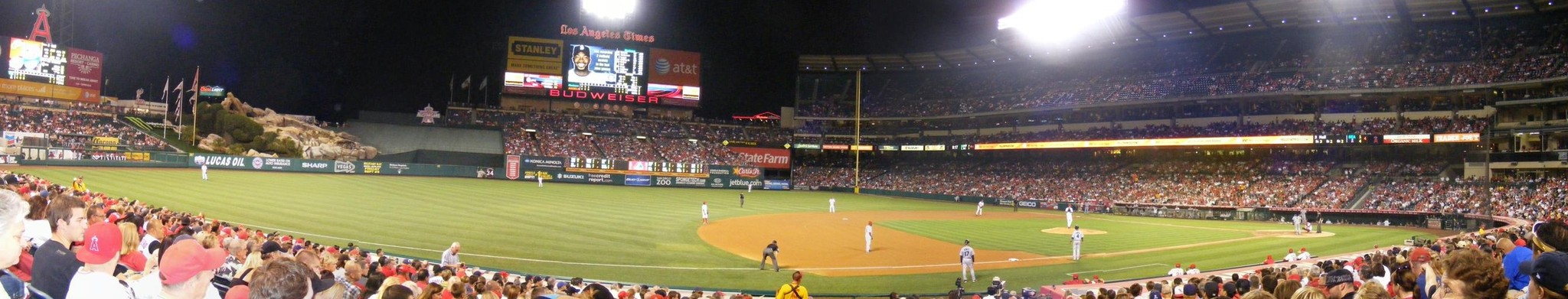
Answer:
[381,256,509,278]
[1075,225,1079,228]
[82,191,117,207]
[261,241,288,254]
[76,221,123,264]
[534,276,544,283]
[4,174,26,185]
[557,281,570,288]
[1519,251,1568,297]
[692,287,701,293]
[222,228,304,243]
[1073,228,1527,287]
[40,190,51,197]
[109,213,127,223]
[309,272,335,293]
[618,291,630,297]
[19,186,32,193]
[568,287,580,294]
[159,239,225,284]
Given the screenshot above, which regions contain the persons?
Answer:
[0,15,1568,299]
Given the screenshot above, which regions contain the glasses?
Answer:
[94,214,107,218]
[1436,280,1475,297]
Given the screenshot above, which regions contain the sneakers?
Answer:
[963,279,977,283]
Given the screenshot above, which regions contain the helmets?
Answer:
[965,240,970,244]
